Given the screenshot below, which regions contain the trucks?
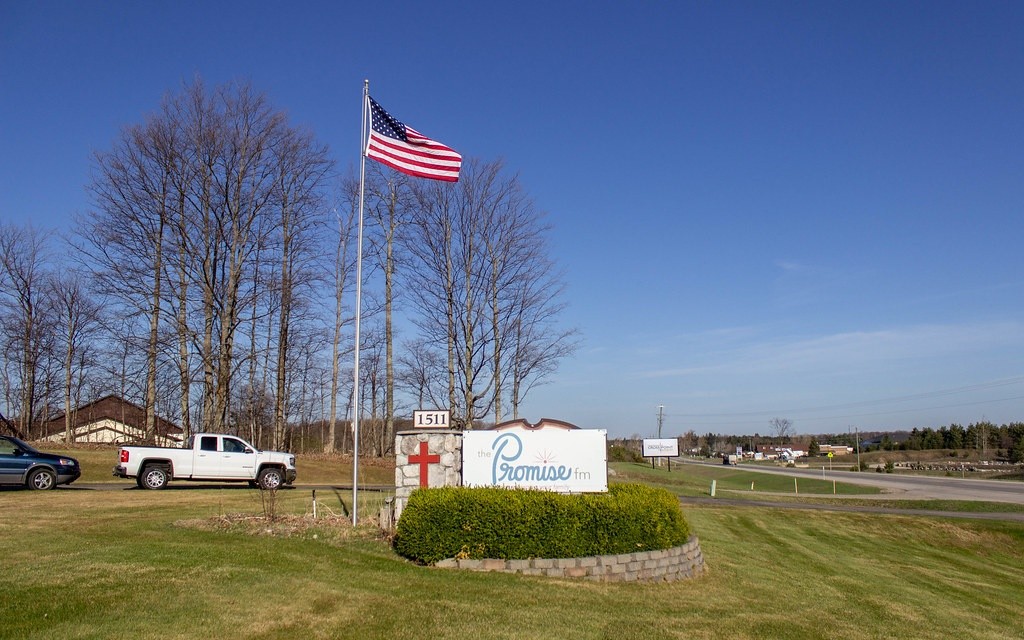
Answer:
[723,455,736,465]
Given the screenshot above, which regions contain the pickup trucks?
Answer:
[112,432,297,489]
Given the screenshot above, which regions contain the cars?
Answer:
[0,435,82,490]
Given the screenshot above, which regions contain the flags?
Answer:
[365,95,463,183]
